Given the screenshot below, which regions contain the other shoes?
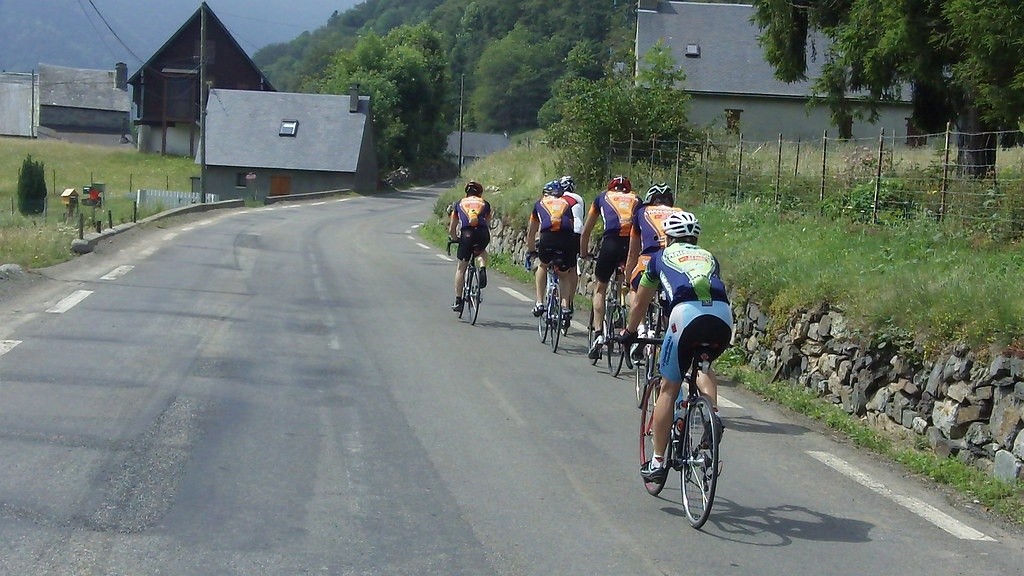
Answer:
[478,266,487,289]
[630,327,647,359]
[452,301,461,312]
[644,331,656,359]
[588,335,604,359]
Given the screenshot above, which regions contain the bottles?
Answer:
[674,386,688,439]
[549,283,553,296]
[646,330,655,355]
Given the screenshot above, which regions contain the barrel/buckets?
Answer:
[82,186,93,205]
[92,182,105,196]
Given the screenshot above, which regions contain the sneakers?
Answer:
[640,460,664,481]
[700,415,723,450]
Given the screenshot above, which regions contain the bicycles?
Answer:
[608,335,724,531]
[446,237,482,325]
[576,253,665,409]
[524,241,573,353]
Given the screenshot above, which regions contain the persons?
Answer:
[449,182,490,311]
[580,176,642,358]
[617,212,735,478]
[527,174,585,314]
[622,182,683,361]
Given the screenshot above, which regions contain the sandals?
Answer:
[562,311,571,325]
[532,304,545,317]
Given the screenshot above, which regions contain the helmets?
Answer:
[543,180,564,199]
[465,181,483,197]
[559,176,576,192]
[609,176,631,194]
[663,211,700,239]
[642,183,674,206]
